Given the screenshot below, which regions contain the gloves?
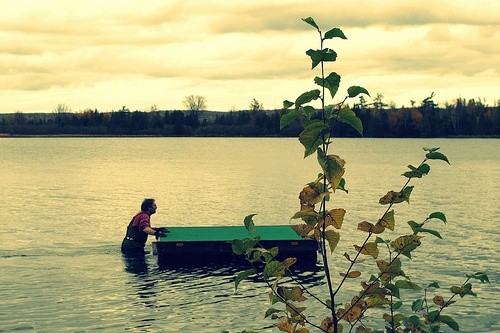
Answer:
[154,231,168,237]
[154,227,171,233]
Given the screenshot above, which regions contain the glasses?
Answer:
[153,204,156,206]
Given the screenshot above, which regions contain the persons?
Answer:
[121,197,172,273]
